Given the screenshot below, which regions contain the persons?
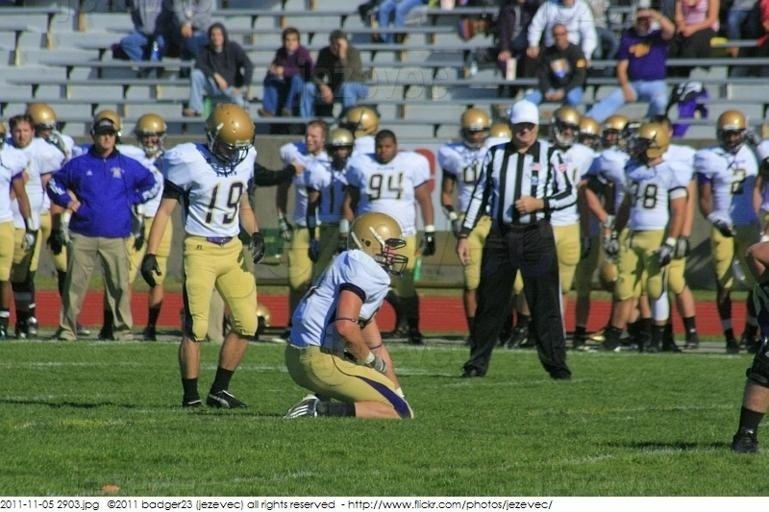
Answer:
[1,1,768,456]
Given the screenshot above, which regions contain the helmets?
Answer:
[0,103,257,169]
[347,212,408,264]
[325,99,747,160]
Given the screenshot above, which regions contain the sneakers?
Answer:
[206,389,249,409]
[0,312,422,343]
[286,394,320,417]
[257,106,292,118]
[183,400,203,408]
[733,427,759,453]
[462,324,757,380]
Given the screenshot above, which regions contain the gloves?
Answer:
[21,214,349,288]
[419,204,738,267]
[359,353,387,374]
